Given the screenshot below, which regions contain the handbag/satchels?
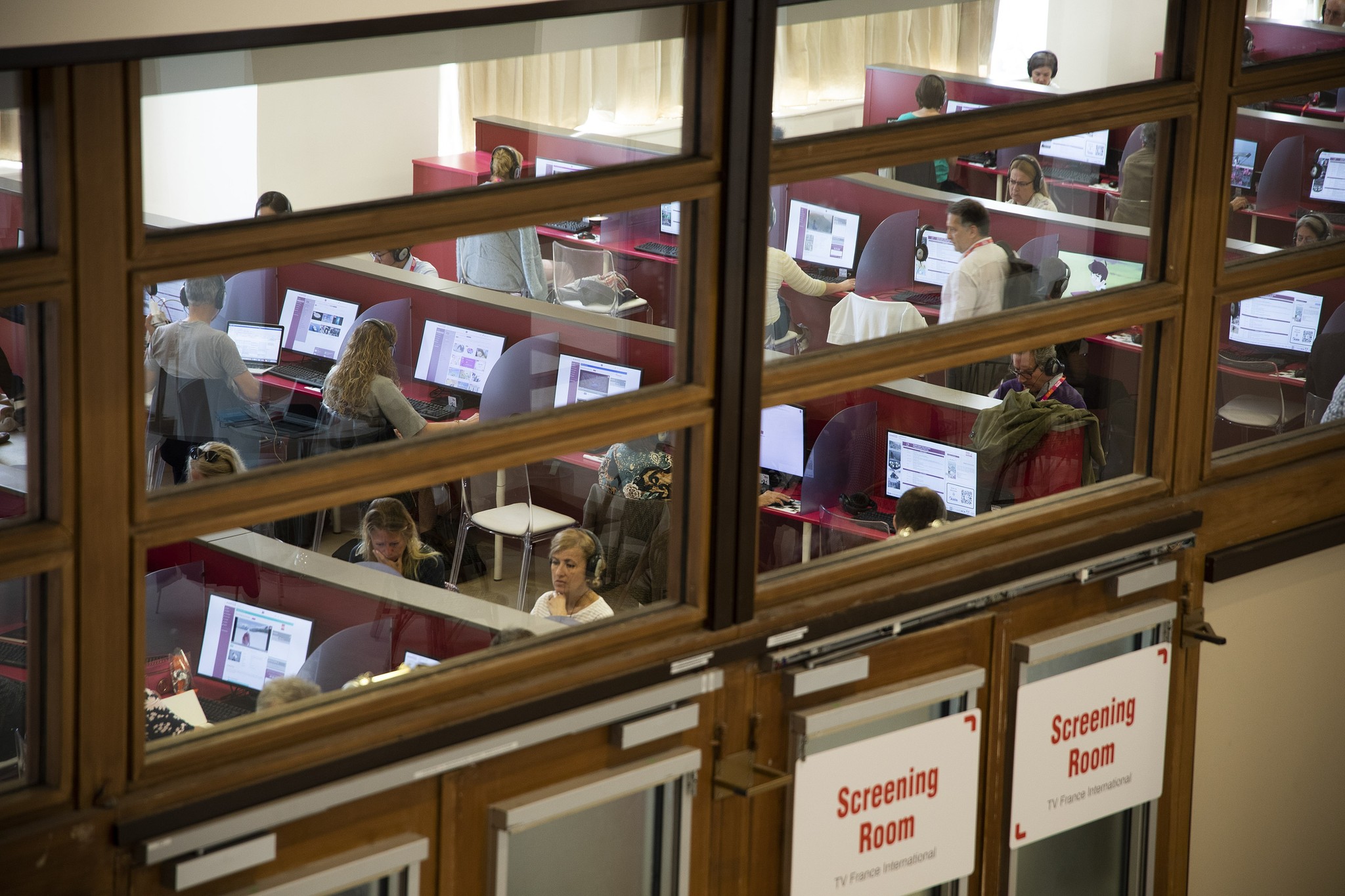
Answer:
[144,651,191,697]
[420,503,487,585]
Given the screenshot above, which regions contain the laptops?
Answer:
[226,320,285,375]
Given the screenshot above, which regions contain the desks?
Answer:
[1271,91,1345,120]
[958,150,1345,245]
[529,217,1141,401]
[240,347,924,583]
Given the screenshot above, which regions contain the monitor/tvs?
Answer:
[759,403,807,488]
[553,352,645,410]
[402,649,440,671]
[412,318,509,410]
[277,287,362,375]
[196,593,315,691]
[489,92,1343,382]
[884,428,980,517]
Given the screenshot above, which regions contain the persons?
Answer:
[0,21,1345,739]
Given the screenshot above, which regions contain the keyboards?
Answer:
[267,364,328,388]
[405,396,461,421]
[196,694,253,722]
[848,508,896,535]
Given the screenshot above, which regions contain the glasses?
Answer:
[1324,9,1345,18]
[1007,178,1034,187]
[1251,45,1255,53]
[1293,233,1318,245]
[189,447,233,472]
[1008,358,1039,379]
[369,249,393,260]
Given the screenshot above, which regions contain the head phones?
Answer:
[361,318,396,358]
[145,282,157,297]
[549,529,606,586]
[838,491,877,516]
[394,243,413,265]
[179,275,226,310]
[654,430,669,442]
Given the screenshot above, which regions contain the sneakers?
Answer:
[796,323,811,351]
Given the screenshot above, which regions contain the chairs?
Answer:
[1102,191,1151,227]
[1004,178,1076,213]
[552,241,653,324]
[815,504,891,562]
[763,288,803,356]
[305,401,409,552]
[1215,354,1307,450]
[457,228,533,299]
[144,368,250,492]
[447,462,582,612]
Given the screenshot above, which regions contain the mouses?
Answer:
[768,499,793,506]
